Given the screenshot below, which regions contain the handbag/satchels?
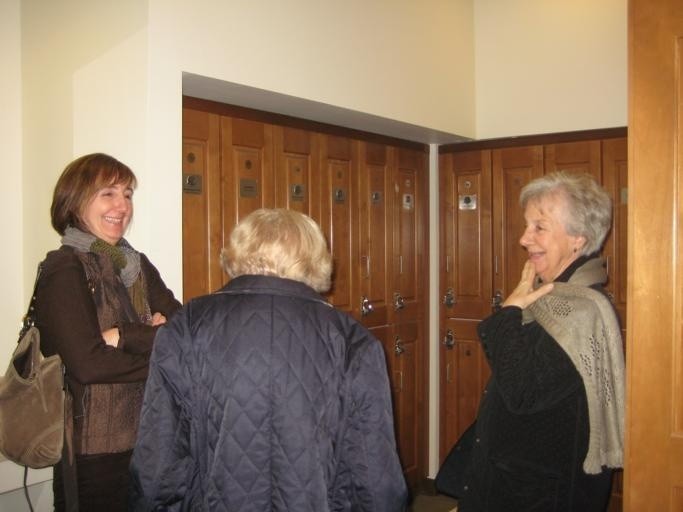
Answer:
[0,325,67,471]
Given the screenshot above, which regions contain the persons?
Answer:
[33,153,182,512]
[128,208,409,512]
[435,169,625,512]
